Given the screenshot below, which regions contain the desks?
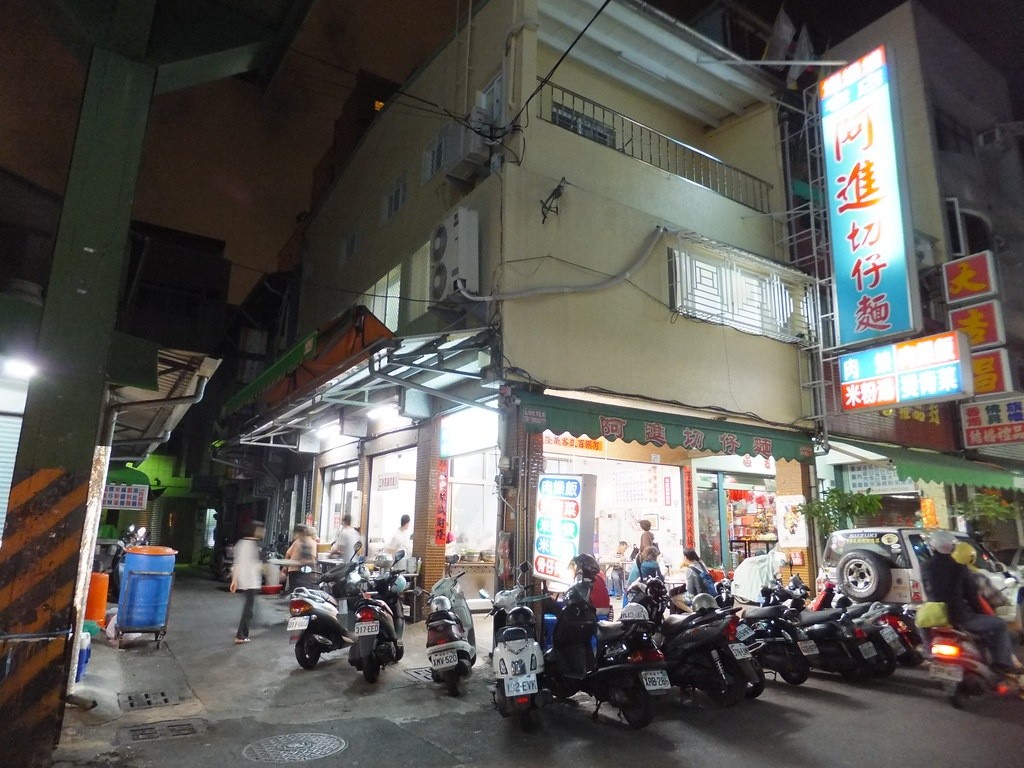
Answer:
[267,559,300,599]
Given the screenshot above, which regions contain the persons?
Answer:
[283,525,320,590]
[918,532,1024,675]
[338,514,360,563]
[230,520,266,643]
[639,520,655,552]
[567,554,610,616]
[683,548,708,602]
[607,541,630,599]
[623,546,664,609]
[390,514,411,556]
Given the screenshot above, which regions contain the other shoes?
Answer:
[992,662,1023,673]
[235,637,251,643]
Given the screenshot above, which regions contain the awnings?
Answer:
[820,435,1024,492]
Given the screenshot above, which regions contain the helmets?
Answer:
[505,605,537,627]
[431,596,451,612]
[389,573,407,593]
[575,552,600,575]
[691,592,720,612]
[619,602,649,620]
[930,532,959,555]
[951,541,976,567]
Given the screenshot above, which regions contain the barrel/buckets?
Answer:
[117,545,178,629]
[84,571,109,628]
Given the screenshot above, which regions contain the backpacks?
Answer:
[690,562,717,597]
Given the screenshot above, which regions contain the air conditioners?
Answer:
[445,105,491,181]
[236,359,265,385]
[238,327,268,354]
[429,204,479,312]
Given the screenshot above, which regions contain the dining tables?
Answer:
[317,553,397,578]
[595,561,686,609]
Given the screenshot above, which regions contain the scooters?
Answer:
[412,553,477,697]
[478,560,548,734]
[285,541,369,672]
[536,564,934,731]
[912,576,1024,712]
[346,548,409,684]
[110,523,152,598]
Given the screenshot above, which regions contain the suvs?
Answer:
[813,523,1024,653]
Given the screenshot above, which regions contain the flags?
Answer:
[764,9,795,71]
[787,25,815,79]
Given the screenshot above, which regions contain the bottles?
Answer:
[417,558,422,574]
[67,632,91,683]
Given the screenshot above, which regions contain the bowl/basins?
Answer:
[403,581,412,590]
[445,549,496,564]
[394,556,417,574]
[262,584,283,594]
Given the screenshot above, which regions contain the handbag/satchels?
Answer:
[553,595,597,645]
